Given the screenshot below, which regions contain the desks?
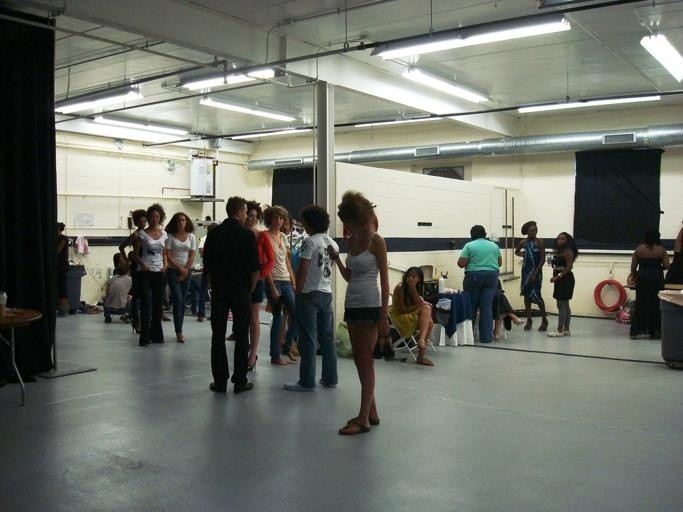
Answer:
[437,289,474,347]
[0,306,43,408]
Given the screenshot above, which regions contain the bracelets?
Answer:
[381,310,388,313]
[558,273,563,278]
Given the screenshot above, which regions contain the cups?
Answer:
[1,291,8,318]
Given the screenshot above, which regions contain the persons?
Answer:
[57,222,69,316]
[547,232,578,337]
[630,228,670,340]
[327,192,390,434]
[665,227,683,284]
[477,278,525,340]
[515,221,548,332]
[457,225,502,342]
[387,267,434,366]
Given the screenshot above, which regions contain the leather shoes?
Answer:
[233,382,253,393]
[208,382,226,393]
[174,332,184,342]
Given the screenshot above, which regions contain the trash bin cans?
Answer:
[658,290,683,370]
[68,265,87,311]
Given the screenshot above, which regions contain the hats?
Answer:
[521,220,537,235]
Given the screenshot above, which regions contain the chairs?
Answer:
[383,306,435,363]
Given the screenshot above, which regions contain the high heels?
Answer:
[523,317,533,331]
[246,353,258,370]
[129,321,140,334]
[536,317,549,332]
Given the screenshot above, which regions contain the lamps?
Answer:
[636,28,683,87]
[197,95,296,125]
[51,84,143,117]
[171,65,274,95]
[366,11,575,62]
[396,65,490,108]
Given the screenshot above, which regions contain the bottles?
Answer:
[437,276,446,293]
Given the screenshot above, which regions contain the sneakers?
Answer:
[317,376,339,388]
[564,330,571,336]
[547,331,564,337]
[512,318,525,328]
[282,380,316,391]
[494,333,505,342]
[104,315,129,324]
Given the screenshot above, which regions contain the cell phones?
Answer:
[327,244,336,257]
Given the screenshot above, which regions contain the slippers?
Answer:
[415,357,433,366]
[337,418,370,435]
[346,416,381,424]
[416,338,428,352]
